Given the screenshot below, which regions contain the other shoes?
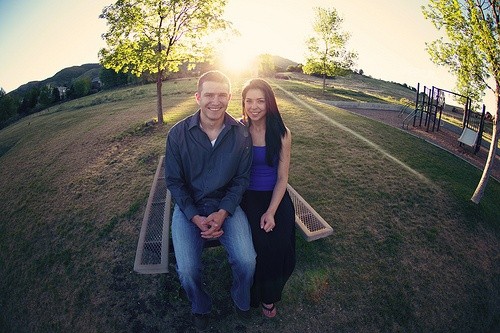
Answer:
[234,301,251,321]
[192,313,208,329]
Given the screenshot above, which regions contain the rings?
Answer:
[271,228,273,232]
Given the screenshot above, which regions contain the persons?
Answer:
[165,70,257,331]
[238,79,296,318]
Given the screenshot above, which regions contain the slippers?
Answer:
[262,304,276,317]
